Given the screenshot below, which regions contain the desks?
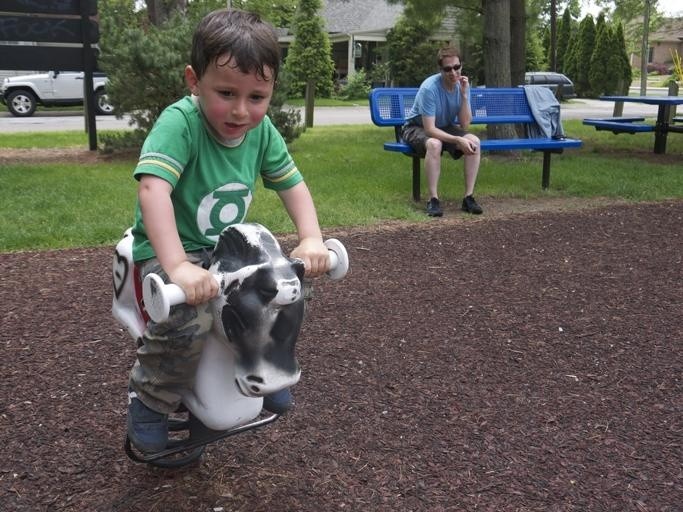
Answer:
[600,95,683,154]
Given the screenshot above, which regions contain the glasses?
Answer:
[442,64,462,73]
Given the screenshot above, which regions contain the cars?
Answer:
[475,71,577,108]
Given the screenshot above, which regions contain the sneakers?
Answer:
[461,194,482,214]
[261,385,294,415]
[123,380,169,455]
[426,197,442,217]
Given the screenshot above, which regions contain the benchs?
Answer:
[583,116,682,153]
[370,84,583,202]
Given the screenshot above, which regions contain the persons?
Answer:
[400,47,483,217]
[128,5,329,453]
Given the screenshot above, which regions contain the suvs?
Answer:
[1,68,118,116]
